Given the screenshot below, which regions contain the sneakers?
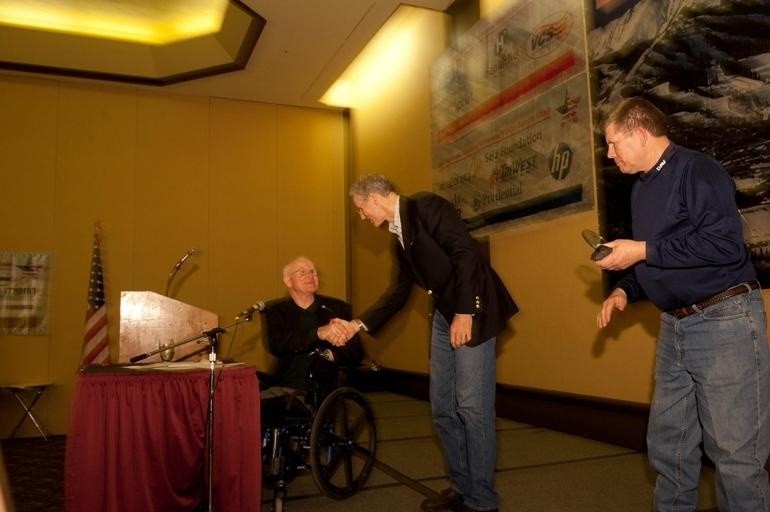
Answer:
[421,488,464,512]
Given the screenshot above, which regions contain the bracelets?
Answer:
[617,288,627,297]
[354,318,363,330]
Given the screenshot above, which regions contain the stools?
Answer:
[1,383,54,441]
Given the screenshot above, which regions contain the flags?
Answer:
[78,243,111,367]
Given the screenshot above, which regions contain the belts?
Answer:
[671,280,760,320]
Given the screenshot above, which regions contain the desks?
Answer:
[76,361,255,512]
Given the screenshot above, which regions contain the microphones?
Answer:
[234,300,265,320]
[169,248,194,276]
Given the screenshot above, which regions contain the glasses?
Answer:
[353,195,370,214]
[291,267,320,280]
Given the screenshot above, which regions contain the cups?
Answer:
[160,339,177,362]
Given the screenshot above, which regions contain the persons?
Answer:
[596,97,770,512]
[258,256,366,456]
[324,172,520,511]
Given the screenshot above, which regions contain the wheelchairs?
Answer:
[262,350,378,500]
